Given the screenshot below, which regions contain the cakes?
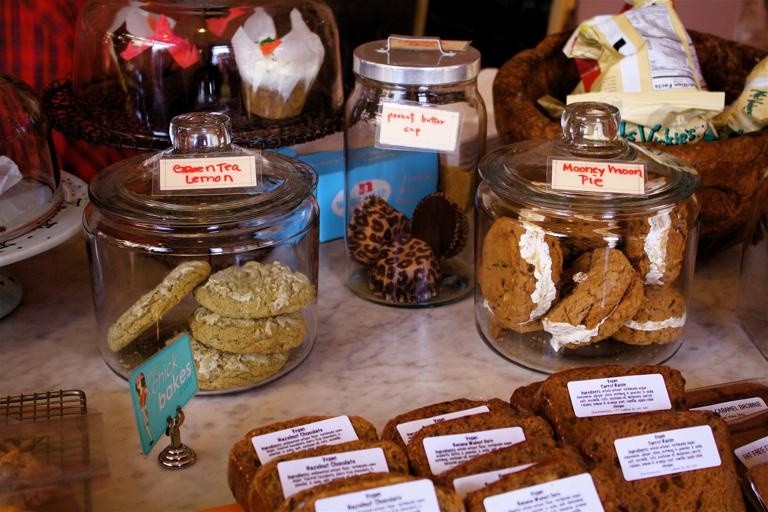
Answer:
[350,190,470,304]
[229,5,325,119]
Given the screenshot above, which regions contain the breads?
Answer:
[226,364,768,512]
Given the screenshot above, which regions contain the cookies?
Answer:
[477,190,691,349]
[107,259,318,393]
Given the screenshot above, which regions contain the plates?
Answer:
[0,167,90,267]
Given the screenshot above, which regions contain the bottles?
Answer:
[80,112,321,397]
[343,34,483,309]
[474,102,705,378]
